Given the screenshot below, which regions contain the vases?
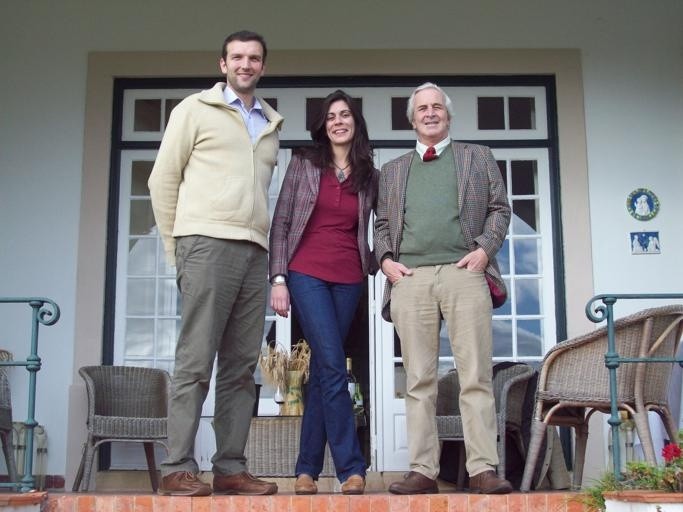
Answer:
[281,368,304,416]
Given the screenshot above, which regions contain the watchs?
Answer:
[270,275,289,287]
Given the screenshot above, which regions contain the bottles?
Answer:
[346,358,356,401]
[352,383,363,415]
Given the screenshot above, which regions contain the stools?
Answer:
[9,421,48,493]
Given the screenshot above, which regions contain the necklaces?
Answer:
[330,160,352,182]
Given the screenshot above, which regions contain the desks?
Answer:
[211,407,364,478]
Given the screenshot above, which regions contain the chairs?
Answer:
[519,304,683,492]
[436,364,537,492]
[0,368,18,493]
[72,365,173,492]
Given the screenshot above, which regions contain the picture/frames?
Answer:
[625,188,661,221]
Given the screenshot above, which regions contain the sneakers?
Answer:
[156,470,213,497]
[388,470,439,494]
[212,469,277,496]
[468,470,514,494]
[294,474,317,494]
[340,474,365,493]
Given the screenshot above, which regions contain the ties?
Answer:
[422,146,437,161]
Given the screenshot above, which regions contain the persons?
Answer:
[634,195,650,216]
[147,30,284,495]
[269,90,382,495]
[373,83,513,493]
[633,235,659,252]
